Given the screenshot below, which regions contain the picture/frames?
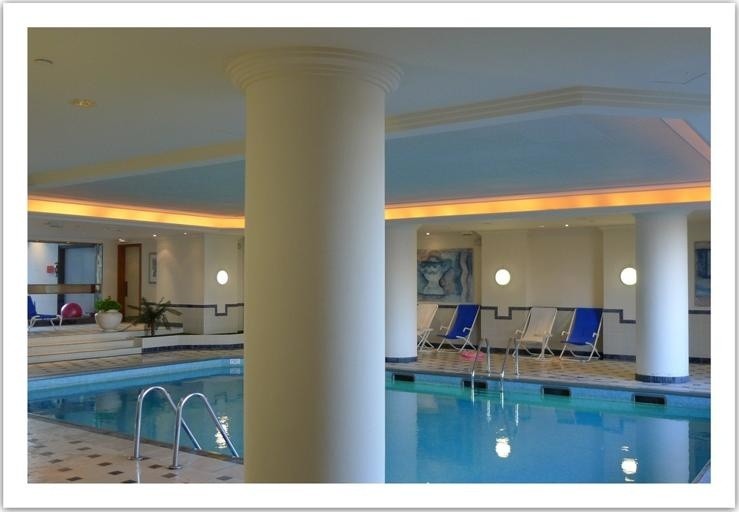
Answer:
[147,252,157,284]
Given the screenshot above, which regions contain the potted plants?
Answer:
[95,297,122,333]
[92,390,121,421]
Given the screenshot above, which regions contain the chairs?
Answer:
[28,295,62,327]
[418,304,439,350]
[513,308,558,360]
[559,308,602,362]
[436,304,481,354]
